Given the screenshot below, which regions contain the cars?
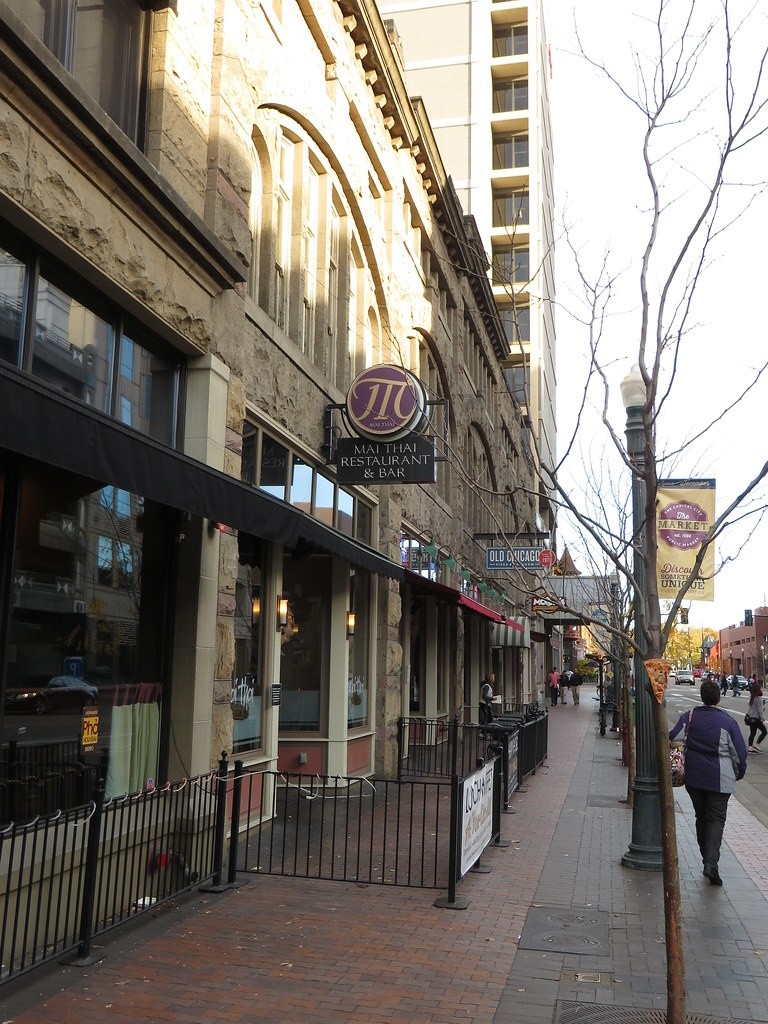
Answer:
[694,668,709,682]
[675,670,696,686]
[669,670,678,677]
[726,674,750,691]
[1,674,101,718]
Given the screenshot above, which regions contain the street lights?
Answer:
[609,567,620,732]
[705,653,708,670]
[760,645,766,687]
[618,356,671,872]
[729,650,733,675]
[684,656,687,671]
[741,648,745,676]
[679,656,683,672]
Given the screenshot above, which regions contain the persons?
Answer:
[559,669,571,704]
[748,685,768,754]
[721,675,728,697]
[547,667,560,706]
[668,681,746,886]
[570,671,584,705]
[478,673,497,740]
[731,673,741,697]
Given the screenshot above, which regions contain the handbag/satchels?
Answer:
[669,708,694,787]
[744,713,750,726]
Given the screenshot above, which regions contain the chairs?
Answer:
[487,725,501,760]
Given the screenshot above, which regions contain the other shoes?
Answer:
[551,703,554,706]
[703,865,722,886]
[564,703,567,704]
[748,746,758,751]
[752,745,761,751]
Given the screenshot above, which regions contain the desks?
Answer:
[492,714,524,728]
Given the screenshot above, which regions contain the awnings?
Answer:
[406,568,500,621]
[1,358,303,547]
[499,614,525,632]
[300,514,404,582]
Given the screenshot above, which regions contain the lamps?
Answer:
[486,580,495,599]
[473,572,487,593]
[494,589,505,603]
[437,558,470,582]
[251,596,260,628]
[400,530,438,559]
[346,611,355,641]
[418,545,458,573]
[276,595,288,632]
